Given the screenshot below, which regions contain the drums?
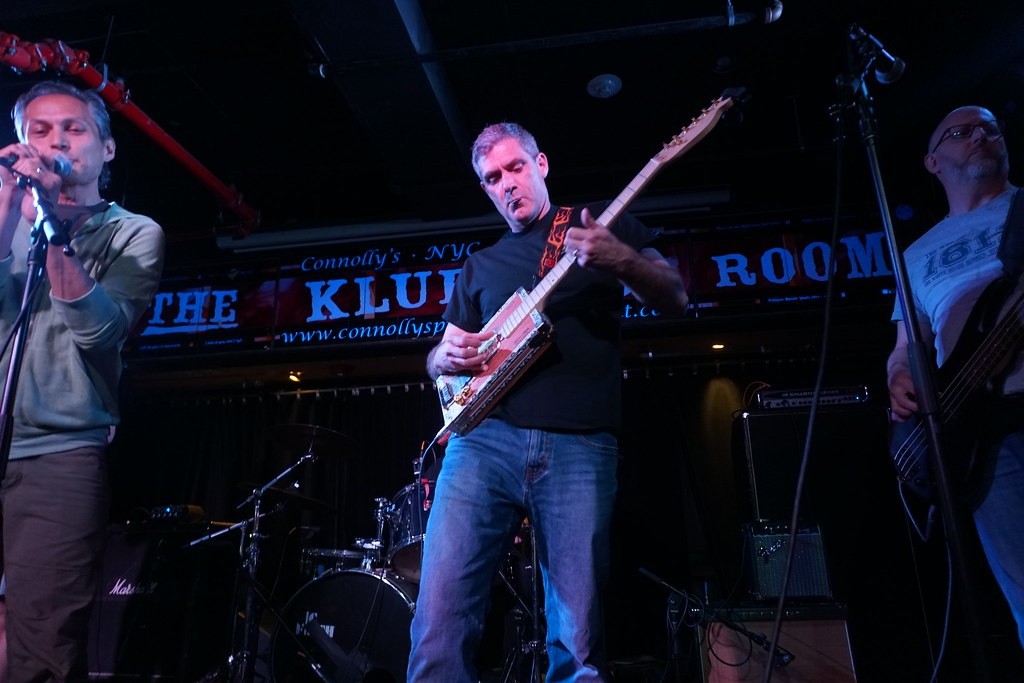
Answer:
[303,547,365,583]
[382,478,439,581]
[268,566,421,683]
[487,550,521,585]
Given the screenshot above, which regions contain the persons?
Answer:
[0,81,165,683]
[406,121,684,683]
[886,106,1024,683]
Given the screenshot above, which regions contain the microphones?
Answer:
[294,525,320,532]
[850,23,905,85]
[8,153,72,176]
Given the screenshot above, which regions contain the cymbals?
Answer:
[240,482,315,499]
[264,422,363,452]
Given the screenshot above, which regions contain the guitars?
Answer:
[891,289,1024,507]
[427,94,735,433]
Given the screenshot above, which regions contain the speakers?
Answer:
[742,520,832,605]
[84,523,235,683]
[696,602,858,683]
[728,406,942,683]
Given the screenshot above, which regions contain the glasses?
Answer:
[931,119,1005,154]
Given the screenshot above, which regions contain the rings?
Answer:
[573,249,579,256]
[574,257,577,261]
